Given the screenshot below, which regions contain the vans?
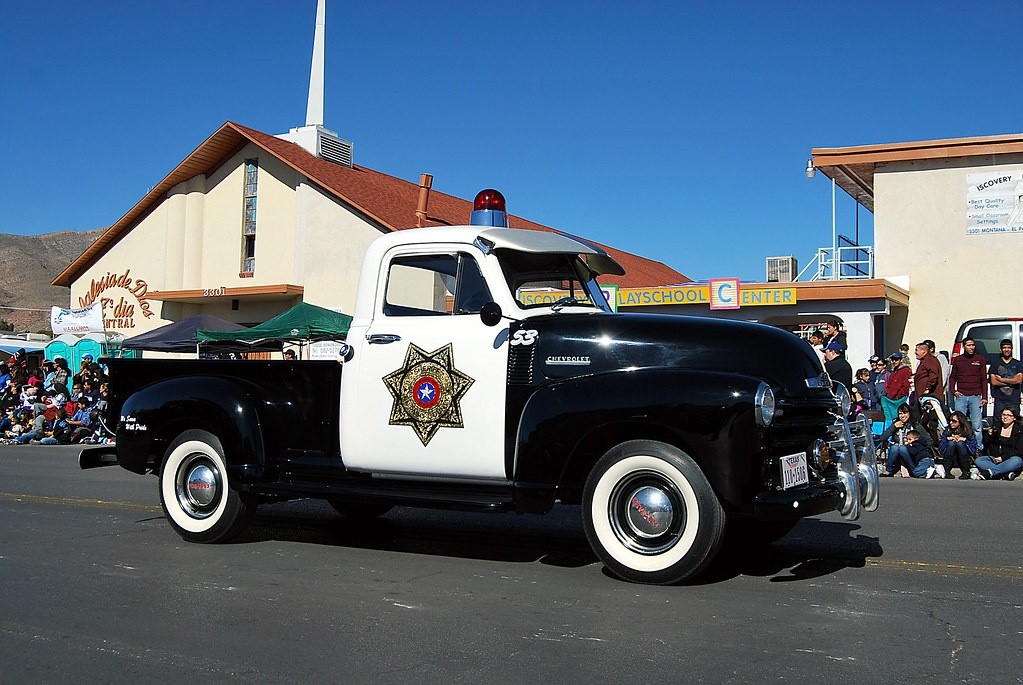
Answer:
[947,317,1023,419]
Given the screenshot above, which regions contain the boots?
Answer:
[944,456,955,479]
[959,454,971,479]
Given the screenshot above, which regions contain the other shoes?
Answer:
[925,466,935,479]
[1005,471,1015,480]
[879,471,894,477]
[977,469,992,480]
[0,435,115,445]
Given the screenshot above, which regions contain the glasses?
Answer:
[876,364,884,366]
[948,419,960,423]
[891,357,899,361]
[1001,414,1013,417]
[72,388,80,390]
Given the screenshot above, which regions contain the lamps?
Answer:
[806,158,817,179]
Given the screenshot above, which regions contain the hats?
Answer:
[868,355,879,361]
[890,352,902,358]
[820,342,843,353]
[82,355,93,360]
[285,349,296,356]
[5,358,16,363]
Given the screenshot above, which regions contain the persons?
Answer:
[809,321,852,394]
[0,348,109,445]
[988,339,1023,416]
[907,430,946,479]
[283,349,295,360]
[974,406,1023,480]
[914,341,950,406]
[949,338,987,456]
[878,403,933,477]
[939,411,977,480]
[847,344,913,457]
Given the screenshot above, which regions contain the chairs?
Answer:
[860,410,886,465]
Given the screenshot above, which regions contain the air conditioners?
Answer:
[765,256,798,283]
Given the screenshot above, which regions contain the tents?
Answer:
[197,302,353,360]
[119,314,284,359]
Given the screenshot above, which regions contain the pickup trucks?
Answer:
[77,198,878,586]
